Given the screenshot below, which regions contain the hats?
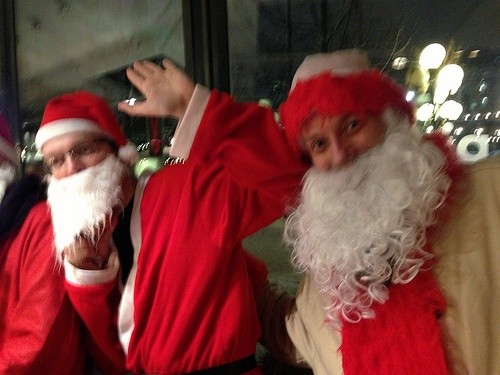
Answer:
[35,90,139,165]
[279,48,415,156]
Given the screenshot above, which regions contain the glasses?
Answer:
[42,137,106,173]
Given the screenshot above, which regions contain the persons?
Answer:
[0,115,86,375]
[34,58,312,375]
[245,48,500,375]
